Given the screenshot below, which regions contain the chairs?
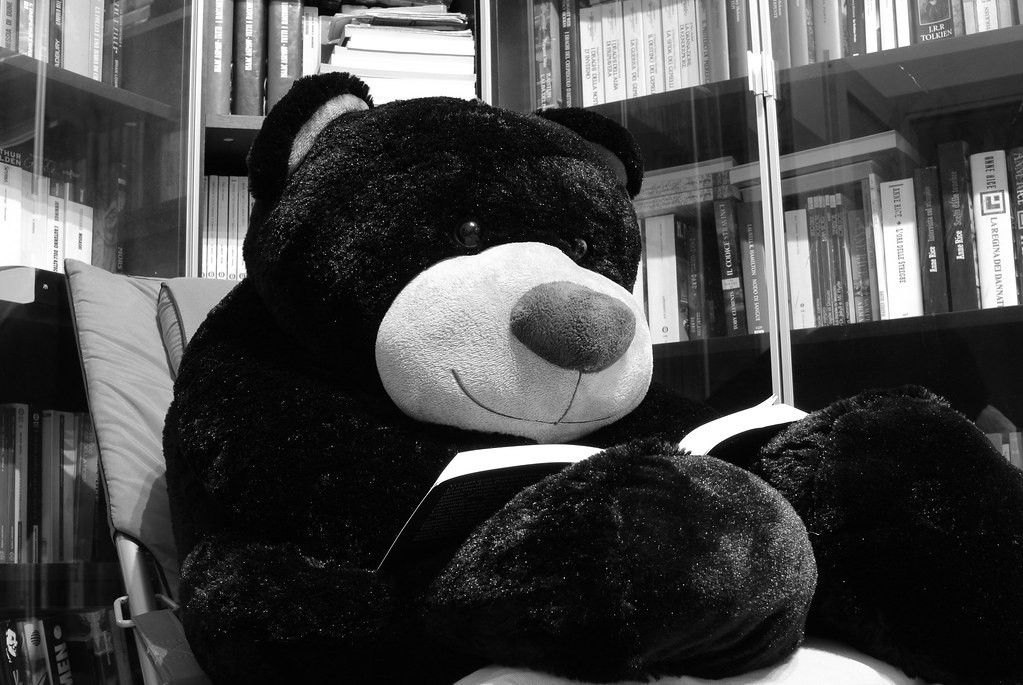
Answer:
[62,252,265,685]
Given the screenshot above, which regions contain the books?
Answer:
[0,0,146,685]
[196,1,1023,475]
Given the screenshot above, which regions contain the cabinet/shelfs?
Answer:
[4,1,1023,685]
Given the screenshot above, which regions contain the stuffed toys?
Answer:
[162,72,1023,685]
